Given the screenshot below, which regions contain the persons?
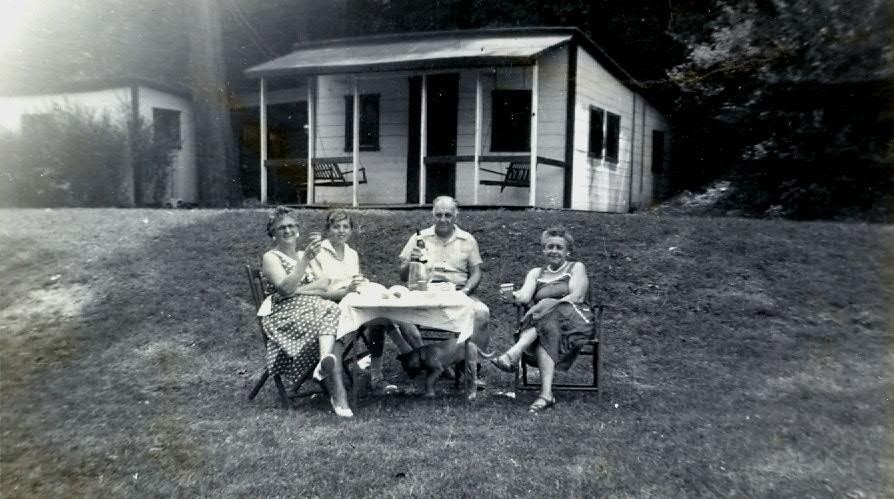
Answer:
[490,228,597,412]
[399,195,490,389]
[258,203,400,418]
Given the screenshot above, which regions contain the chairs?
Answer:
[242,264,372,409]
[511,282,604,399]
[419,324,462,392]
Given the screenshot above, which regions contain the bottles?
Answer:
[415,224,428,264]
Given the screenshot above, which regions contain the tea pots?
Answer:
[409,261,435,291]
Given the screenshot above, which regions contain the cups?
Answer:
[500,283,514,306]
[308,232,320,247]
[418,281,427,291]
[352,274,364,282]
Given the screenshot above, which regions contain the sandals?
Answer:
[490,352,518,374]
[528,393,556,413]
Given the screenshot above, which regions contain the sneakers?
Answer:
[330,394,353,419]
[313,354,337,382]
[476,376,487,389]
[370,378,399,396]
